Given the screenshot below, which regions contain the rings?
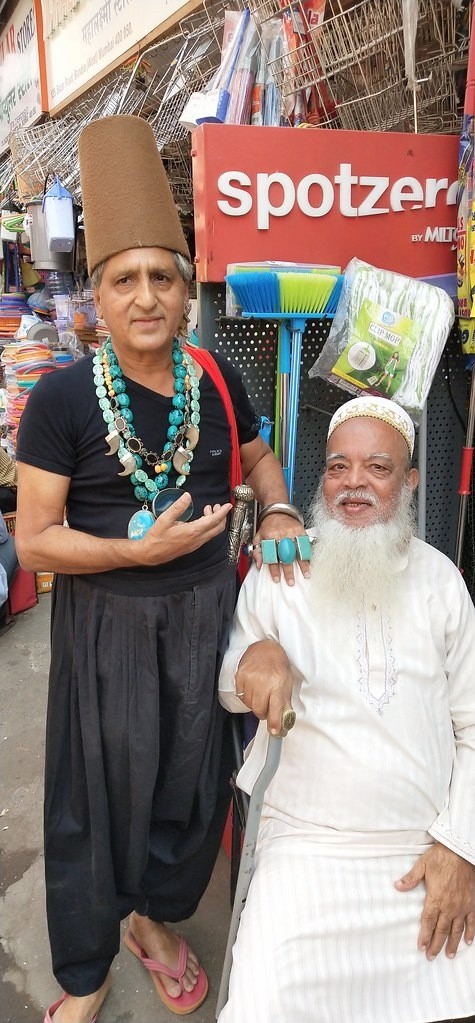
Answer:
[236,692,245,697]
[253,535,311,564]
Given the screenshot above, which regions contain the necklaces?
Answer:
[93,335,201,541]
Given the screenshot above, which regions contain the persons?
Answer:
[15,118,309,1023]
[213,397,475,1023]
[0,447,17,630]
[375,351,401,394]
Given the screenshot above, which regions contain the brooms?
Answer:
[224,264,347,503]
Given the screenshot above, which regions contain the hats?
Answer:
[326,395,415,461]
[79,115,192,278]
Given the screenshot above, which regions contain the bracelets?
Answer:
[258,502,304,527]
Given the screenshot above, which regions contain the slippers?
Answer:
[43,992,98,1023]
[125,926,208,1015]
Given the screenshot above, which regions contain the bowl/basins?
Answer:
[5,376,18,427]
[7,395,29,461]
[17,342,47,353]
[52,347,74,369]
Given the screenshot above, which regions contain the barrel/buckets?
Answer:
[26,200,83,272]
[1,345,18,375]
[53,295,70,320]
[12,356,56,399]
[16,350,57,364]
[54,320,67,335]
